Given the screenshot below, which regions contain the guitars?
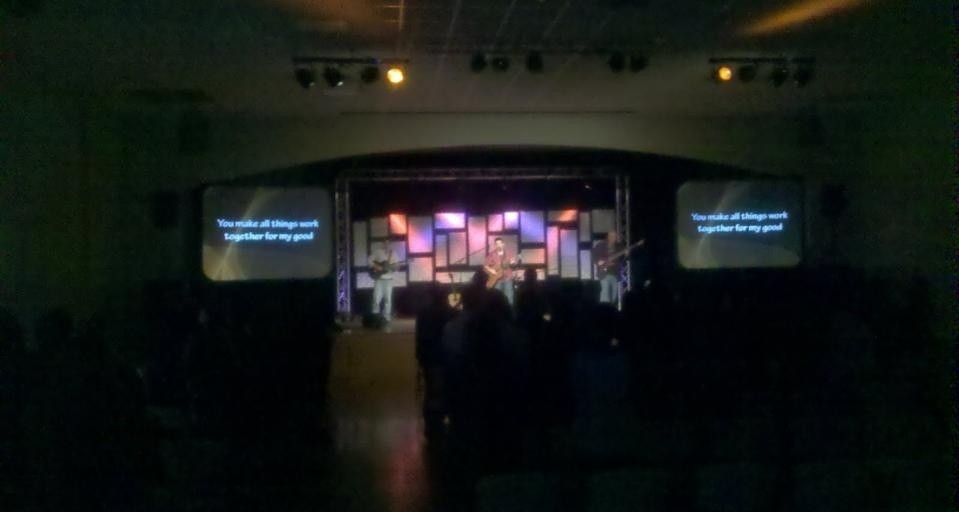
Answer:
[481,254,522,290]
[448,271,463,310]
[596,239,645,279]
[369,257,415,278]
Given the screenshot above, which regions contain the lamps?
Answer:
[288,56,406,97]
[464,42,655,75]
[709,58,815,92]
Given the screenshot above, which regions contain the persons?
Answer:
[592,227,624,306]
[1,271,339,511]
[483,238,514,305]
[414,267,959,511]
[367,237,396,324]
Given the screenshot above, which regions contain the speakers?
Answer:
[151,191,178,228]
[367,313,386,329]
[821,183,846,218]
